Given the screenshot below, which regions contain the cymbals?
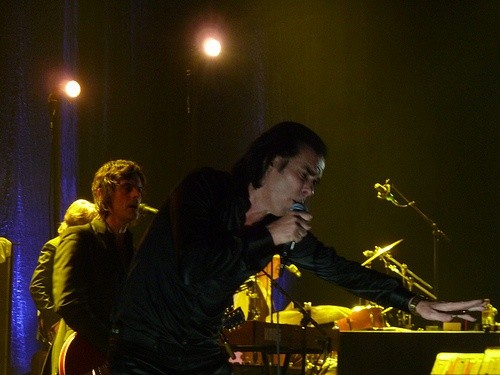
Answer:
[264,304,353,327]
[360,237,405,267]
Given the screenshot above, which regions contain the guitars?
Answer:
[36,311,62,342]
[58,305,247,375]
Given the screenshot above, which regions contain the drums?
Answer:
[334,306,387,333]
[380,306,405,328]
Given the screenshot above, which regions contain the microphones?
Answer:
[138,202,159,216]
[282,204,307,257]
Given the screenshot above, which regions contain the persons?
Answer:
[110,120,489,375]
[271,262,292,312]
[51,160,148,375]
[29,199,103,375]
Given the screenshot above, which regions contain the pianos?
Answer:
[217,320,340,375]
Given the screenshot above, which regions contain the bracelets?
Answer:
[410,297,422,316]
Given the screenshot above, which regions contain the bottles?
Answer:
[482,299,494,328]
